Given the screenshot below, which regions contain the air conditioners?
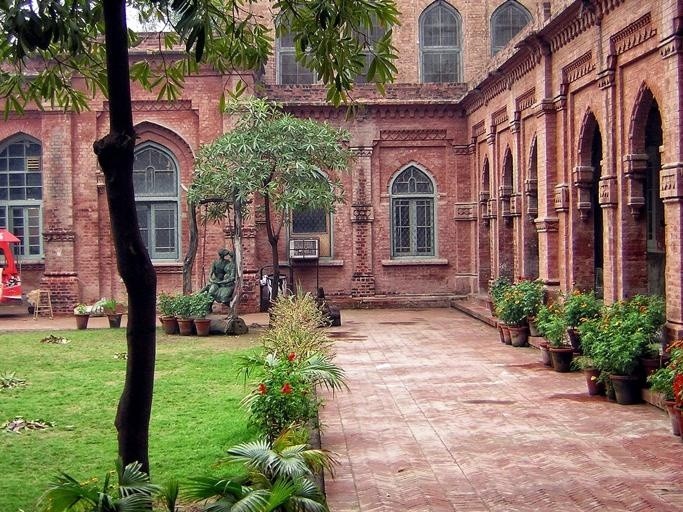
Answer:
[290,239,319,260]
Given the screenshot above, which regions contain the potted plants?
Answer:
[74,304,90,329]
[486,275,683,441]
[158,289,216,336]
[99,299,123,328]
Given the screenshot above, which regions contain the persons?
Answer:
[212,251,236,305]
[197,248,236,300]
